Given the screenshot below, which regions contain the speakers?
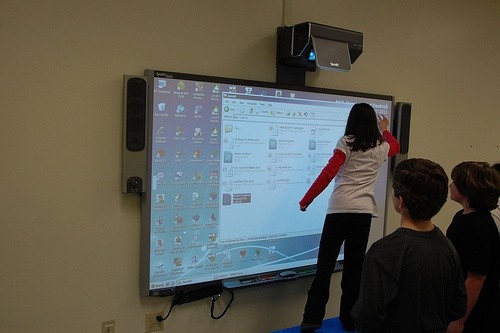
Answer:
[121,74,149,194]
[391,102,412,173]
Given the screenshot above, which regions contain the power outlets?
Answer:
[144,310,164,331]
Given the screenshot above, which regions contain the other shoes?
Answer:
[300,316,323,332]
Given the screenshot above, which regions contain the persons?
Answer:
[350,158,467,332]
[447,161,500,332]
[299,100,400,332]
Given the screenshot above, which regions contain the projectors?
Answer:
[291,21,364,73]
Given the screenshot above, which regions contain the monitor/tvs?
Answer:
[139,69,395,298]
